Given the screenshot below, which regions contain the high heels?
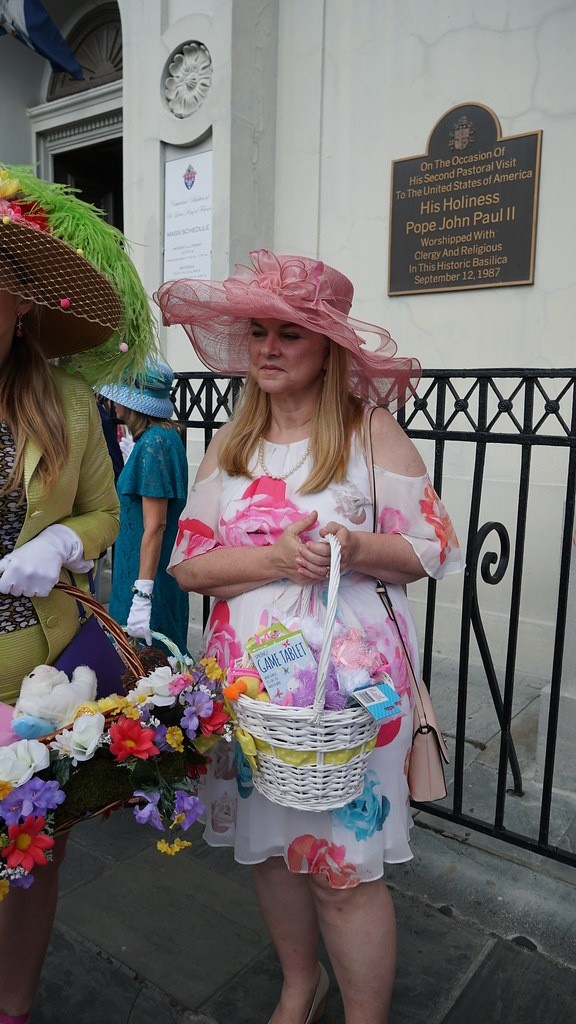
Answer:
[265,961,330,1024]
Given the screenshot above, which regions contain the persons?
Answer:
[0,287,120,1023]
[166,315,459,1024]
[91,350,188,666]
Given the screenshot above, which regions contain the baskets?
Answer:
[219,533,383,811]
[36,583,195,842]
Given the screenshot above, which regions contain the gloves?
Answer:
[1,523,94,600]
[125,579,154,647]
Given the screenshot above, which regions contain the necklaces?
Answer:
[259,420,312,481]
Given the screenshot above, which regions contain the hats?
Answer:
[93,360,175,419]
[0,216,119,358]
[155,250,422,414]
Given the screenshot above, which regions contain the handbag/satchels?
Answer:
[409,678,450,803]
